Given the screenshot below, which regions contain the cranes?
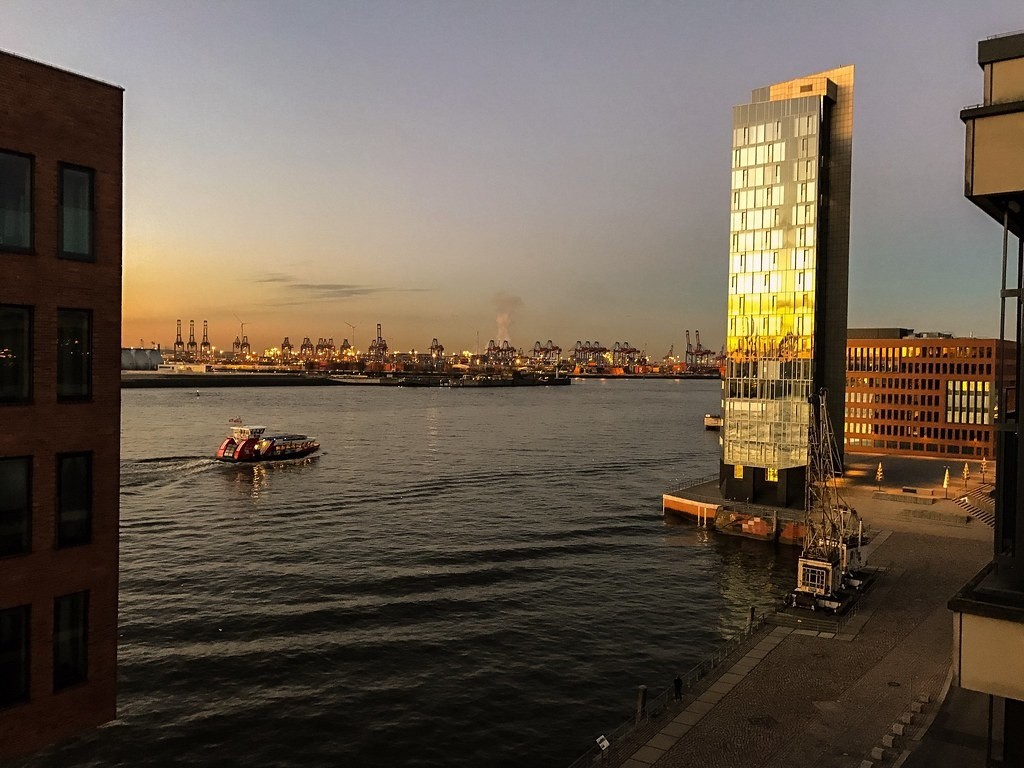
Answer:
[339,338,351,354]
[301,337,314,355]
[574,340,610,364]
[685,329,716,366]
[613,341,640,367]
[368,324,388,355]
[174,319,184,356]
[430,338,444,358]
[187,320,197,359]
[281,337,294,354]
[345,321,356,346]
[315,337,335,354]
[534,339,559,358]
[200,320,211,358]
[487,339,516,358]
[232,313,251,355]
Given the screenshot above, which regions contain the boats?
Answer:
[215,425,320,463]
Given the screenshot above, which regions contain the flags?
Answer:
[234,417,242,423]
[229,417,238,422]
